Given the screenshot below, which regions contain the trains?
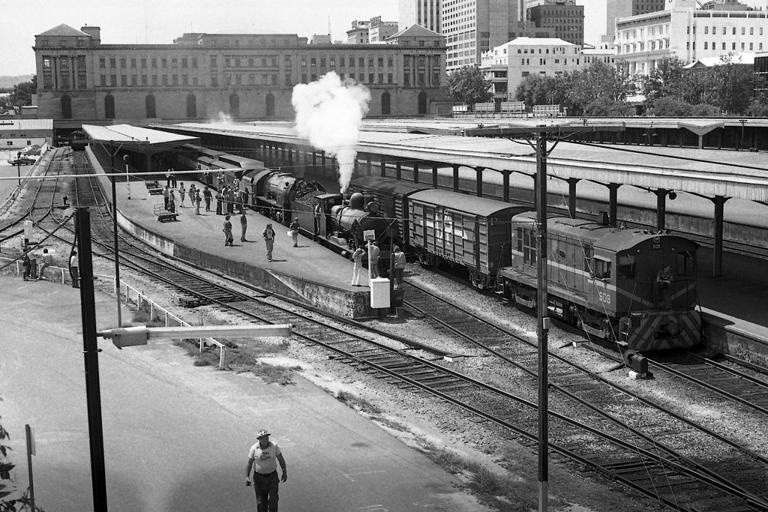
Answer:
[159,141,403,274]
[343,175,705,354]
[56,129,70,147]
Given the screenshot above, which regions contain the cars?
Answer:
[7,144,41,166]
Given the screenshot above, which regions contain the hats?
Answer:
[256,429,272,439]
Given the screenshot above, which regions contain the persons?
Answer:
[288,216,300,248]
[69,250,80,288]
[221,214,233,246]
[243,427,287,512]
[21,246,53,283]
[214,182,249,216]
[164,167,212,215]
[351,240,406,289]
[239,210,247,242]
[262,223,275,262]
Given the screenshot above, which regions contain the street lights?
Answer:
[72,207,293,512]
